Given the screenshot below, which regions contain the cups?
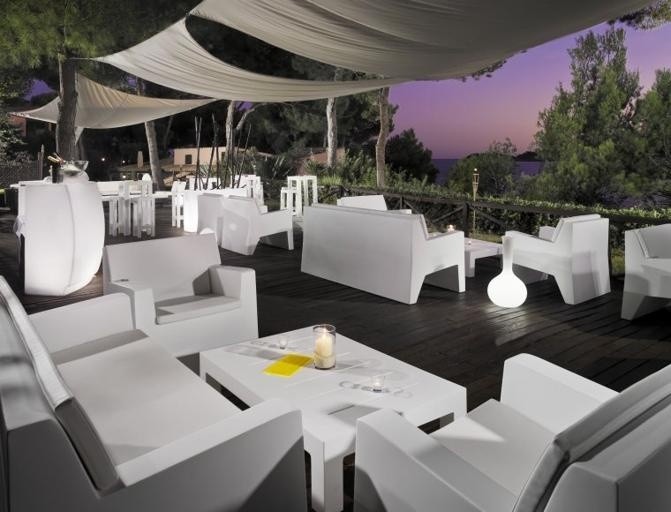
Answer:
[312,323,337,371]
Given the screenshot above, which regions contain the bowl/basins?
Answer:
[60,159,89,177]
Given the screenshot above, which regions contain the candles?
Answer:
[315,327,333,357]
[448,225,454,233]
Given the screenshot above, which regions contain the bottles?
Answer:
[47,152,67,165]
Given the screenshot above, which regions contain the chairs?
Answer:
[620,223,671,321]
[336,195,413,215]
[505,214,612,306]
[183,190,225,234]
[103,233,259,358]
[220,195,295,255]
[352,353,671,512]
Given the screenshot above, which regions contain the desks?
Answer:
[13,180,105,297]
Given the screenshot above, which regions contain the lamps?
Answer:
[486,236,528,309]
[471,168,480,202]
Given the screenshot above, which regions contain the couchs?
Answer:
[300,203,467,306]
[0,274,308,512]
